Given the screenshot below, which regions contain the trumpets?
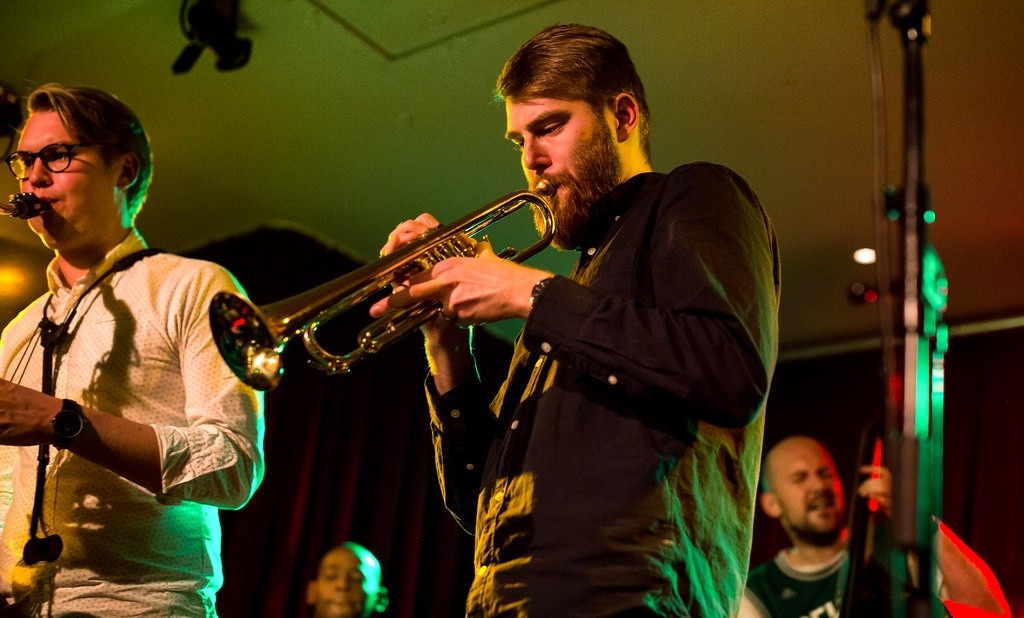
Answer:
[209,177,557,391]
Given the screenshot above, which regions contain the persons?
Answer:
[304,544,392,618]
[0,79,263,618]
[368,23,778,618]
[739,436,995,618]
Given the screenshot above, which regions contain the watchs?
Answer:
[49,399,86,449]
[529,278,553,311]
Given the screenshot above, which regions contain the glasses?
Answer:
[4,142,97,181]
